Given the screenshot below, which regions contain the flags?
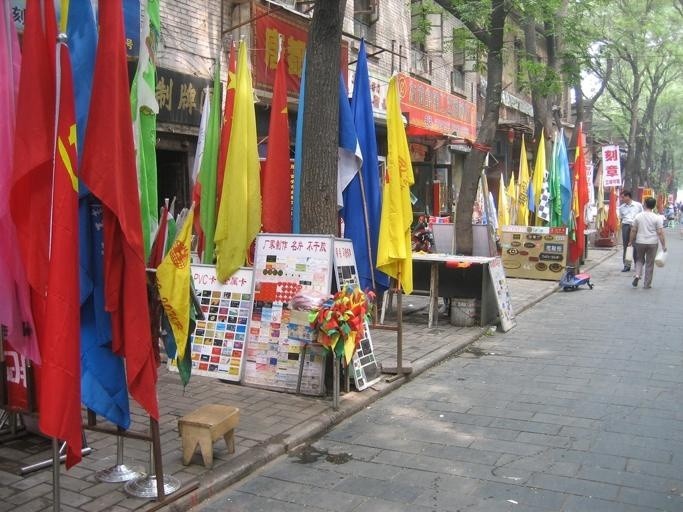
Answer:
[595,175,619,234]
[484,126,590,265]
[338,40,417,297]
[2,0,162,470]
[191,38,306,285]
[148,204,205,396]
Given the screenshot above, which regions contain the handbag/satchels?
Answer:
[625,244,634,266]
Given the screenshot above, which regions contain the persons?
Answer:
[627,196,667,290]
[618,191,643,273]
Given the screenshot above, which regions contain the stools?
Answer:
[297,340,350,410]
[178,406,237,471]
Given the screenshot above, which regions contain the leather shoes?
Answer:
[622,266,630,272]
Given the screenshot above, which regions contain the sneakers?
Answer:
[632,275,640,286]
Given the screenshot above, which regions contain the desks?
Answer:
[379,255,517,331]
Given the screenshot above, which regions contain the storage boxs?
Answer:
[288,310,317,343]
